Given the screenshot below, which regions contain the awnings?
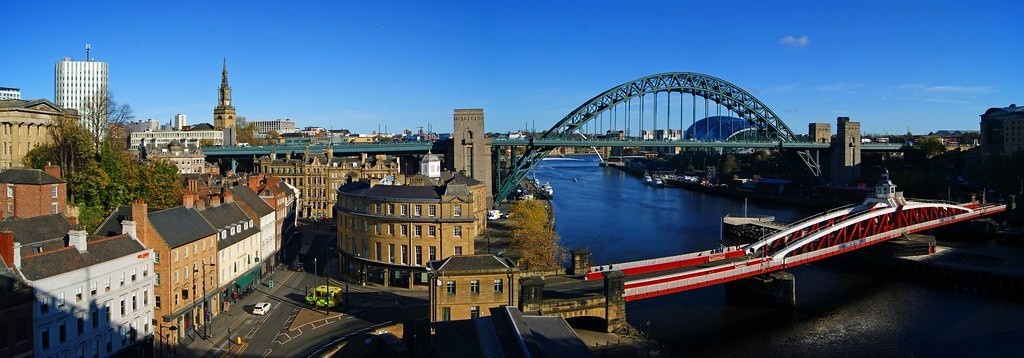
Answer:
[236,273,257,288]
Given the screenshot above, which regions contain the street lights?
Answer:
[159,324,177,358]
[202,264,215,339]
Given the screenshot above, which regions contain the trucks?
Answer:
[305,285,342,308]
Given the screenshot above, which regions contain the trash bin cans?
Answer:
[269,279,274,288]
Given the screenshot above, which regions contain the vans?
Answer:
[489,210,503,220]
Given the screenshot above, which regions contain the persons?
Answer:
[709,243,731,255]
[233,292,238,304]
[594,263,613,271]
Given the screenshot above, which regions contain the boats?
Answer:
[541,183,554,199]
[644,176,662,186]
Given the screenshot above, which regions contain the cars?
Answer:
[253,302,271,316]
